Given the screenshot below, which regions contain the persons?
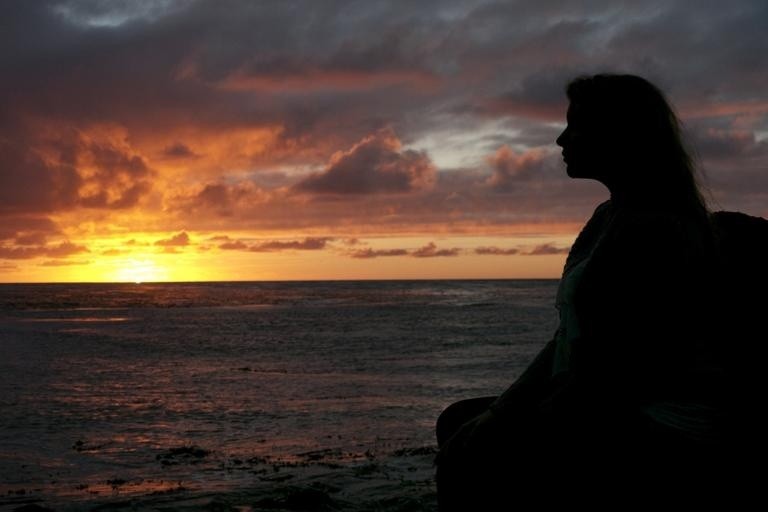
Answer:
[432,73,725,511]
